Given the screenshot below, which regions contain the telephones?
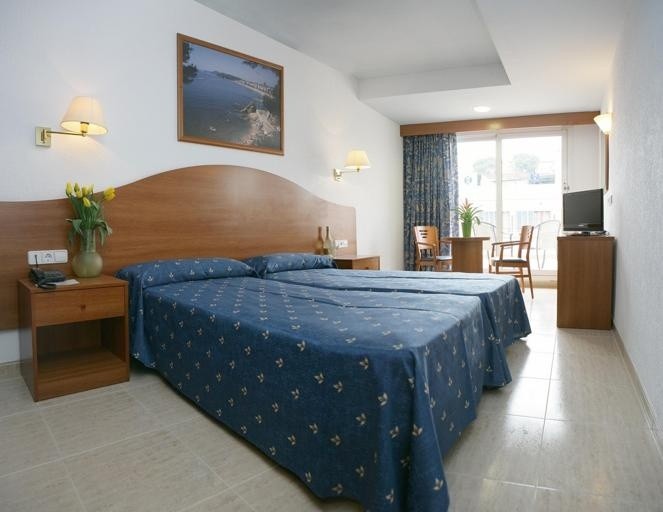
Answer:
[29,267,65,283]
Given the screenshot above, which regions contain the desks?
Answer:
[442,237,490,273]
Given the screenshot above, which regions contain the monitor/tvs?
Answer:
[563,188,603,235]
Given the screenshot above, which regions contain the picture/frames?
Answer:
[177,31,284,156]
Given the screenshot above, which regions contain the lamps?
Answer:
[593,113,610,136]
[35,96,108,147]
[334,151,371,182]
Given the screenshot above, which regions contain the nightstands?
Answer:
[16,272,130,403]
[333,255,380,270]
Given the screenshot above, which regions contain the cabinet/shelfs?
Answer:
[557,233,615,330]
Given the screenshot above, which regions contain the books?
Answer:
[95,340,121,359]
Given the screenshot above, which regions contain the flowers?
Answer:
[64,181,116,248]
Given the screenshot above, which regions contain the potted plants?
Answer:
[450,197,484,237]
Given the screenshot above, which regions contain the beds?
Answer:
[242,252,531,348]
[114,257,482,511]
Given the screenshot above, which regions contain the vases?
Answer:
[71,228,104,278]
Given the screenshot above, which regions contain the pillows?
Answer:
[118,256,257,289]
[242,252,333,275]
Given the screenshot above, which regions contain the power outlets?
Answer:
[40,250,55,265]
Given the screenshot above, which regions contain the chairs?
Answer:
[518,220,560,270]
[489,225,535,299]
[472,221,516,270]
[412,225,452,271]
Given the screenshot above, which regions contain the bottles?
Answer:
[314,226,323,255]
[323,226,333,257]
